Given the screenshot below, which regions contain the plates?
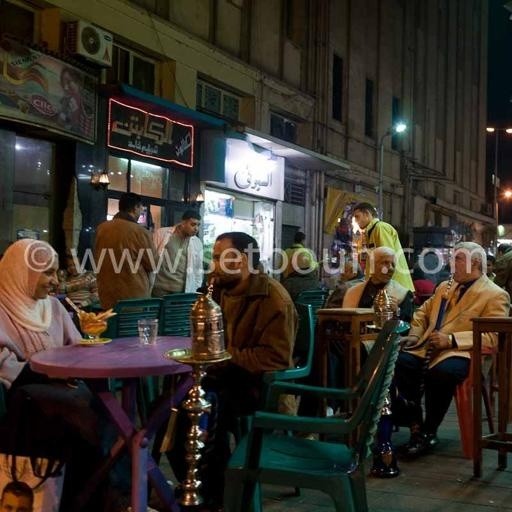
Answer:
[75,338,112,346]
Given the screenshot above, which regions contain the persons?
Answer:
[0,240,151,511]
[299,247,413,442]
[61,254,97,297]
[487,256,505,289]
[411,267,435,306]
[322,262,359,328]
[486,244,512,299]
[155,232,297,483]
[354,203,417,300]
[282,231,316,277]
[279,253,326,359]
[155,211,203,296]
[96,192,158,311]
[389,242,510,461]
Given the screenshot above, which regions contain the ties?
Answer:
[455,285,467,305]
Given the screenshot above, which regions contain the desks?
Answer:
[29,336,229,512]
[470,313,512,482]
[317,307,396,467]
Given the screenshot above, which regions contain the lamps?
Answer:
[90,169,112,193]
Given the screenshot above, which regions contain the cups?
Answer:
[137,319,159,349]
[80,319,108,340]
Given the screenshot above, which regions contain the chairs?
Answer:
[296,292,331,315]
[102,299,163,403]
[249,300,313,411]
[457,368,493,461]
[225,322,411,512]
[158,294,205,337]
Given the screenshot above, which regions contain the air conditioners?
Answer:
[69,17,114,72]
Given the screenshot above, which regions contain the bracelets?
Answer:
[447,334,452,348]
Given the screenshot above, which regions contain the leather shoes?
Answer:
[396,432,440,461]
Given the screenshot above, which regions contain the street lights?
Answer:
[485,126,511,219]
[377,121,407,221]
